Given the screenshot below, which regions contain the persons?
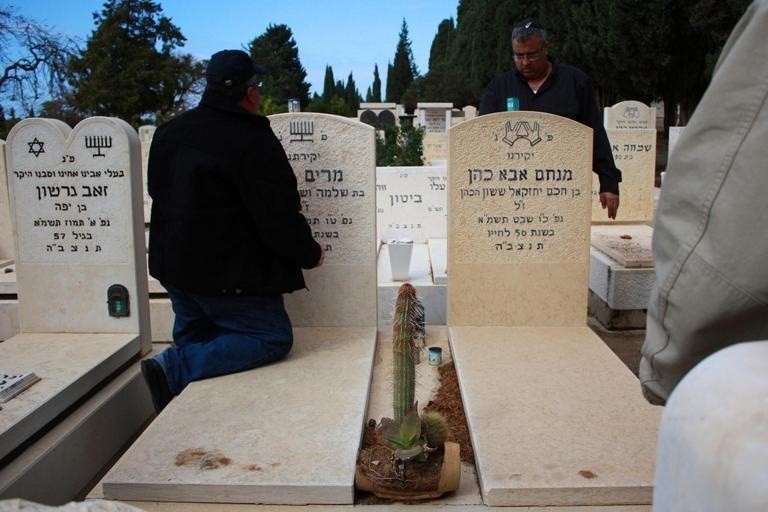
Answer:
[636,2,767,407]
[138,46,325,416]
[479,20,621,220]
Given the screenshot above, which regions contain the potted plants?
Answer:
[355,281,463,506]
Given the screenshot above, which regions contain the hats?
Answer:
[206,50,267,86]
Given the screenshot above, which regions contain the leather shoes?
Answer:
[141,358,175,415]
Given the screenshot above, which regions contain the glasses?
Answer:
[257,80,263,88]
[511,53,540,61]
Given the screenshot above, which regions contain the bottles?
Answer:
[427,345,443,365]
